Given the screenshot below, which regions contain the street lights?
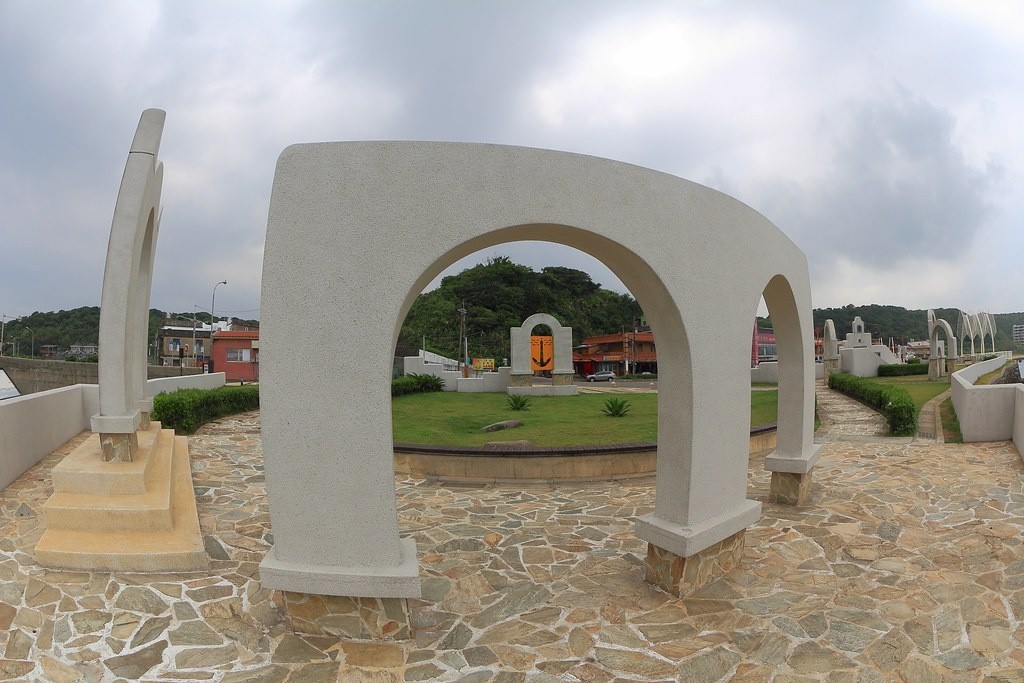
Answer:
[26,326,34,360]
[0,314,21,358]
[210,280,227,373]
[176,315,202,368]
[10,335,17,357]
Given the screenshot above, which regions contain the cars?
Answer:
[587,370,618,382]
